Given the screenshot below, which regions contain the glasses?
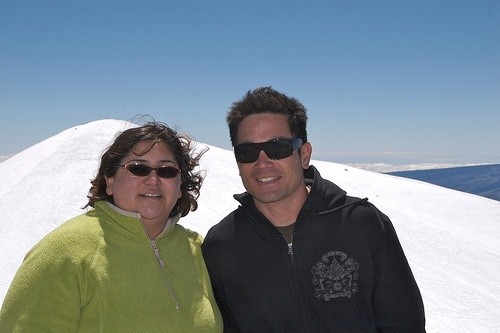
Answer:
[235,138,304,164]
[114,161,182,178]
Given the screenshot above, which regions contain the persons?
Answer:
[0,113,223,333]
[202,86,426,333]
